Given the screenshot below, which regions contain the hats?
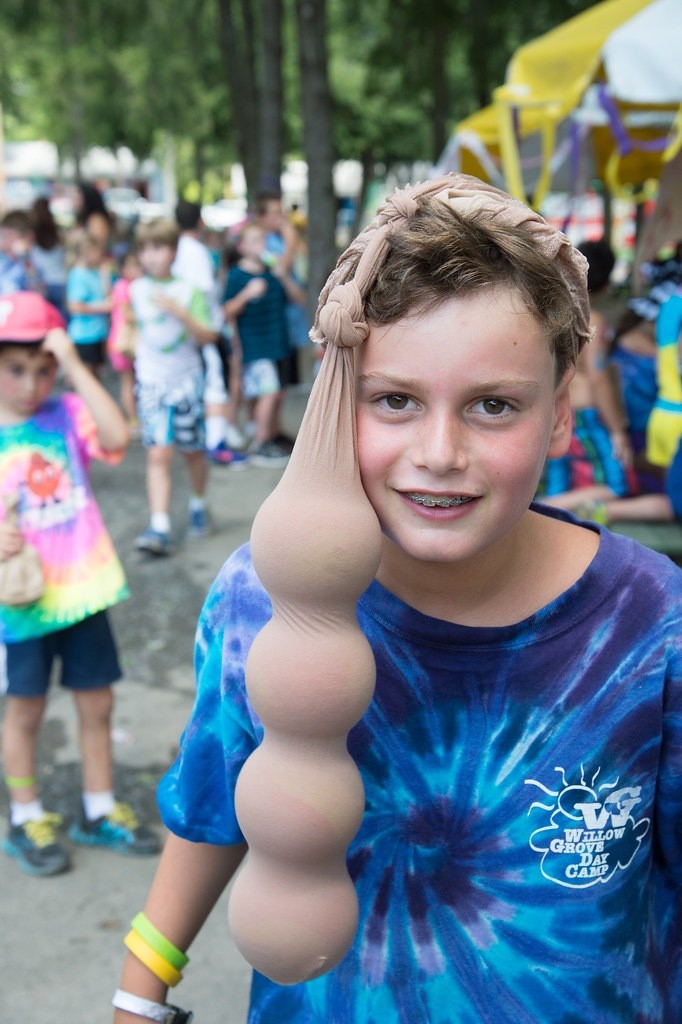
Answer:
[0,290,67,342]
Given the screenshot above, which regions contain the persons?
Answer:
[110,205,680,1023]
[576,263,678,524]
[530,239,634,515]
[0,293,163,876]
[0,192,311,557]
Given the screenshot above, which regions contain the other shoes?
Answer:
[200,422,297,471]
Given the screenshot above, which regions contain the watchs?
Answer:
[113,988,194,1024]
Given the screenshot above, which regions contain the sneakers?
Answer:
[6,815,71,875]
[135,530,175,556]
[70,801,161,853]
[186,502,215,537]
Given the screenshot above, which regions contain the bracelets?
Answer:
[122,928,183,987]
[130,913,189,971]
